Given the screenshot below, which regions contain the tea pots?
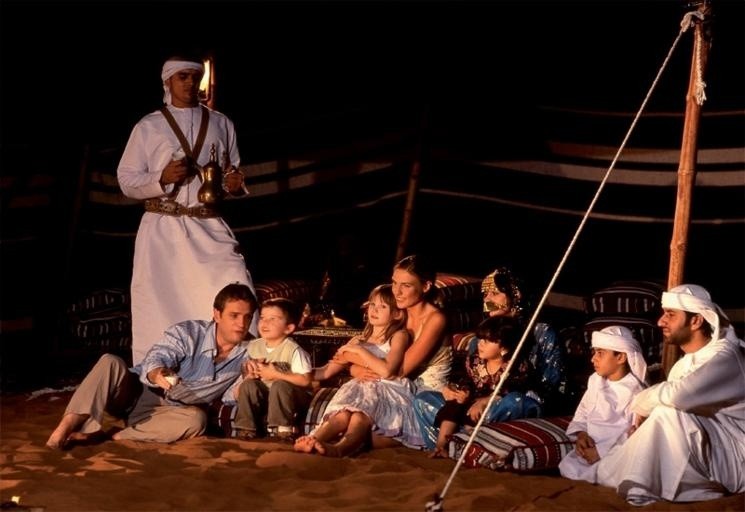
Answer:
[196,142,227,205]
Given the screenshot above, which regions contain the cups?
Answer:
[170,151,187,162]
[164,375,181,387]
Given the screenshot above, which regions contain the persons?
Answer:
[114,52,259,366]
